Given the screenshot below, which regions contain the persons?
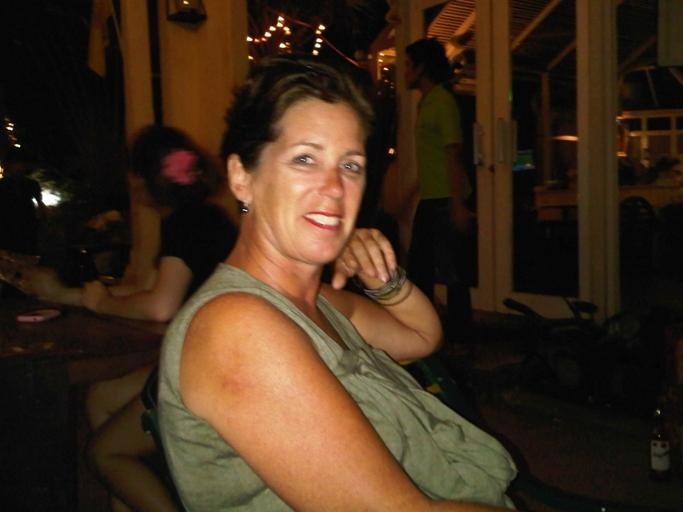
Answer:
[153,56,522,512]
[394,34,478,332]
[0,148,62,252]
[26,126,239,512]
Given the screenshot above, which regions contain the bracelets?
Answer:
[350,267,413,307]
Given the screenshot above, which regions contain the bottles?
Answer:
[647,407,673,480]
